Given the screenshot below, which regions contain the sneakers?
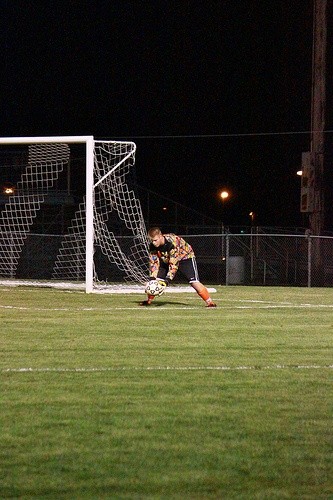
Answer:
[205,303,216,307]
[143,301,153,305]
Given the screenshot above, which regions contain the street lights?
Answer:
[220,190,231,258]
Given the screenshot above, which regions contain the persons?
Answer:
[138,227,218,308]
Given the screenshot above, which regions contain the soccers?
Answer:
[146,280,161,295]
